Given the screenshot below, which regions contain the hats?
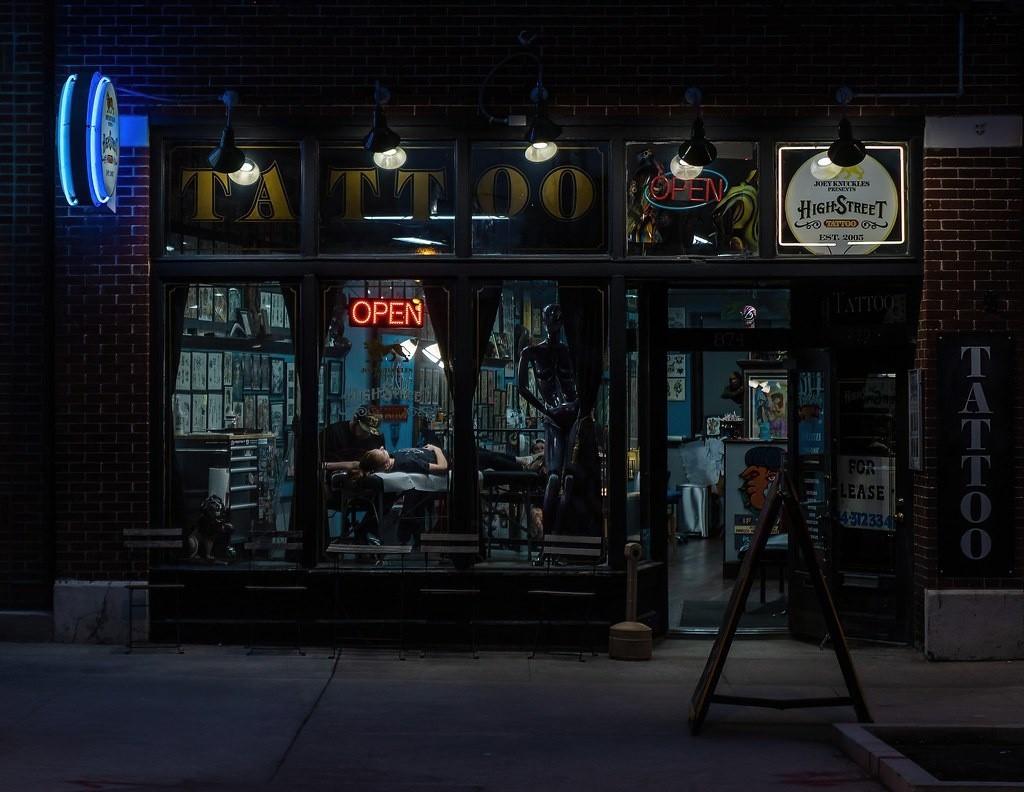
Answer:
[528,438,546,451]
[357,404,383,436]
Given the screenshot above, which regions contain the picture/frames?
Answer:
[268,400,287,443]
[284,425,295,480]
[269,356,286,396]
[742,371,791,440]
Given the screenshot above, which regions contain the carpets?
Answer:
[680,601,790,628]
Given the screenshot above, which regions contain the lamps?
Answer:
[811,117,864,181]
[522,114,562,162]
[671,109,712,182]
[363,91,408,169]
[207,95,262,186]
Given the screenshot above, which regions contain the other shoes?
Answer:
[528,452,545,472]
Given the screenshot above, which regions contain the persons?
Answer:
[518,301,579,533]
[320,404,386,545]
[531,438,547,453]
[344,444,546,496]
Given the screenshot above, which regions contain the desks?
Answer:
[668,489,683,555]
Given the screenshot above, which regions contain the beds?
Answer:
[333,466,512,545]
